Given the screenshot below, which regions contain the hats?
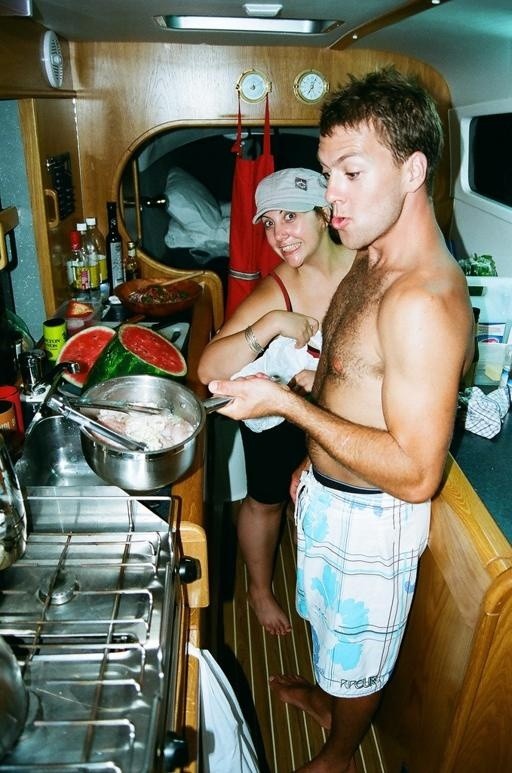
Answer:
[252,167,333,225]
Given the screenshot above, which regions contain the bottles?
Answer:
[19,349,44,391]
[67,202,139,300]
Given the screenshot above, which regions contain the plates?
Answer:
[113,277,202,317]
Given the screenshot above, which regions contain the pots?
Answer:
[78,375,234,492]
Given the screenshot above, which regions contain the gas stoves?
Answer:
[0,484,200,768]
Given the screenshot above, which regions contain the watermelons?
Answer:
[56,325,115,389]
[80,323,187,393]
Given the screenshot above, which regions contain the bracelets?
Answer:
[244,325,265,355]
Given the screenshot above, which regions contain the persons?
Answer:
[197,168,359,638]
[208,60,478,772]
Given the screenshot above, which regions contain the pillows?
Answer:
[164,164,221,244]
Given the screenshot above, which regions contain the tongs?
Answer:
[45,391,173,456]
